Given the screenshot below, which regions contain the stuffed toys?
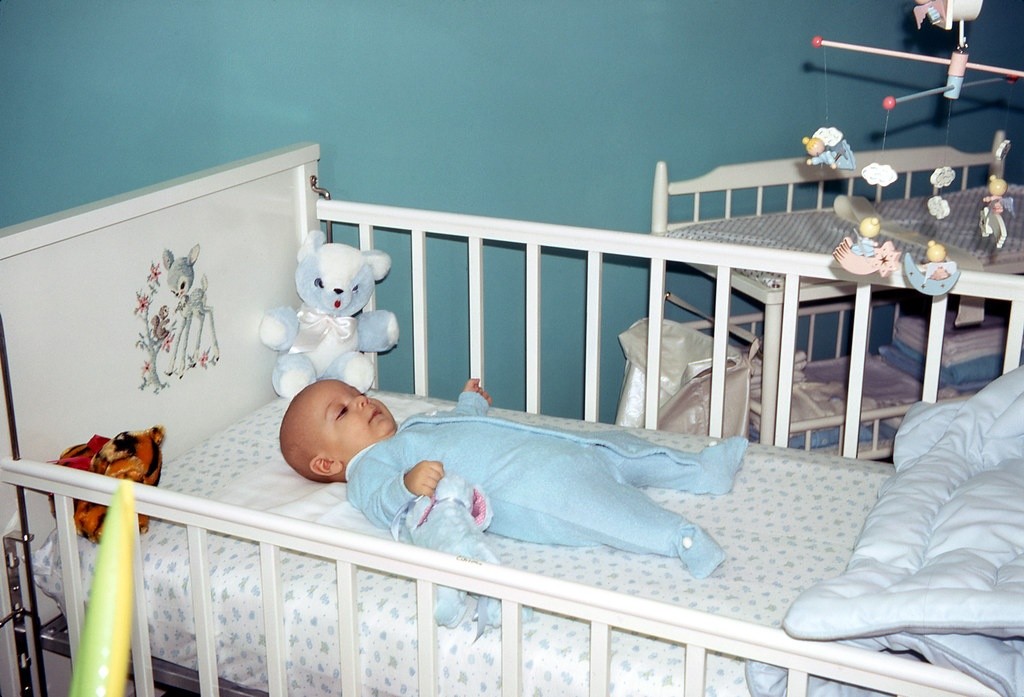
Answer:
[388,476,532,643]
[260,230,400,400]
[47,425,168,547]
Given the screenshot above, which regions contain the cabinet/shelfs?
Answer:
[643,127,1024,462]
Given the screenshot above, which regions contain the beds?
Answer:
[0,137,1024,697]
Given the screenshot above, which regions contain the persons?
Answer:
[850,217,880,258]
[910,0,943,23]
[921,240,949,280]
[279,379,748,579]
[983,175,1008,213]
[802,136,856,171]
